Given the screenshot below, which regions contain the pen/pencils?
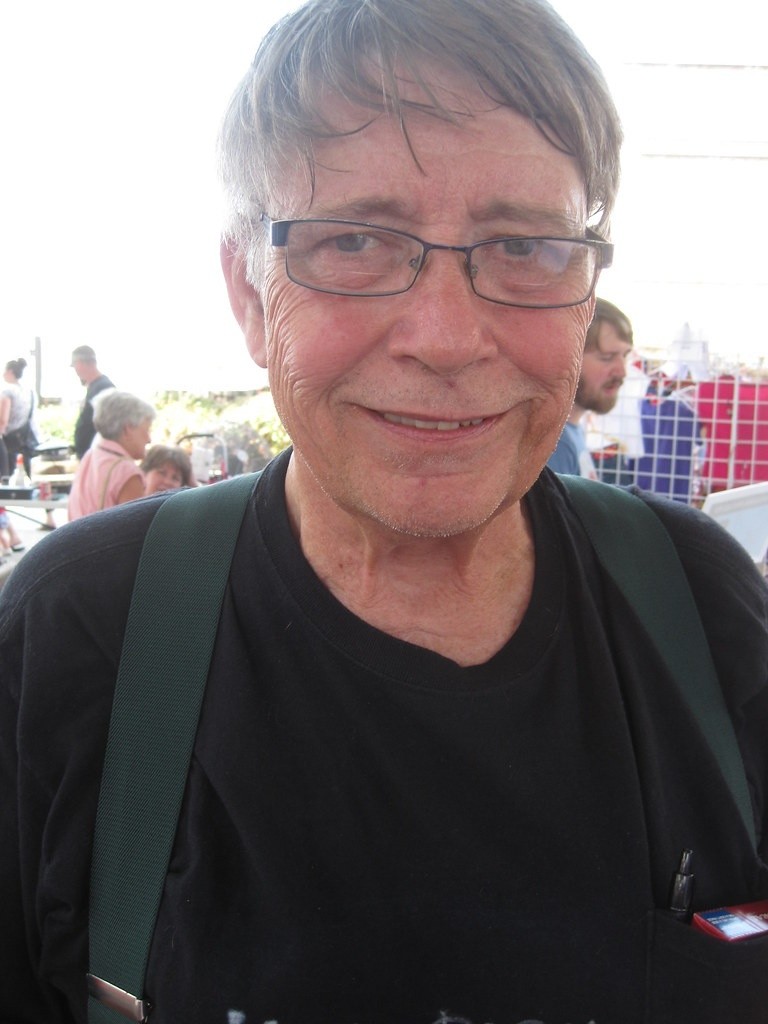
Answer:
[671,847,695,921]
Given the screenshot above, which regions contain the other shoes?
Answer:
[1,545,25,555]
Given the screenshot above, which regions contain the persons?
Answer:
[1,0,766,1024]
[1,295,635,563]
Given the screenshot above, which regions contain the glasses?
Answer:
[255,207,614,310]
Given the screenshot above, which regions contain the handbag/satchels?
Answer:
[4,421,39,465]
